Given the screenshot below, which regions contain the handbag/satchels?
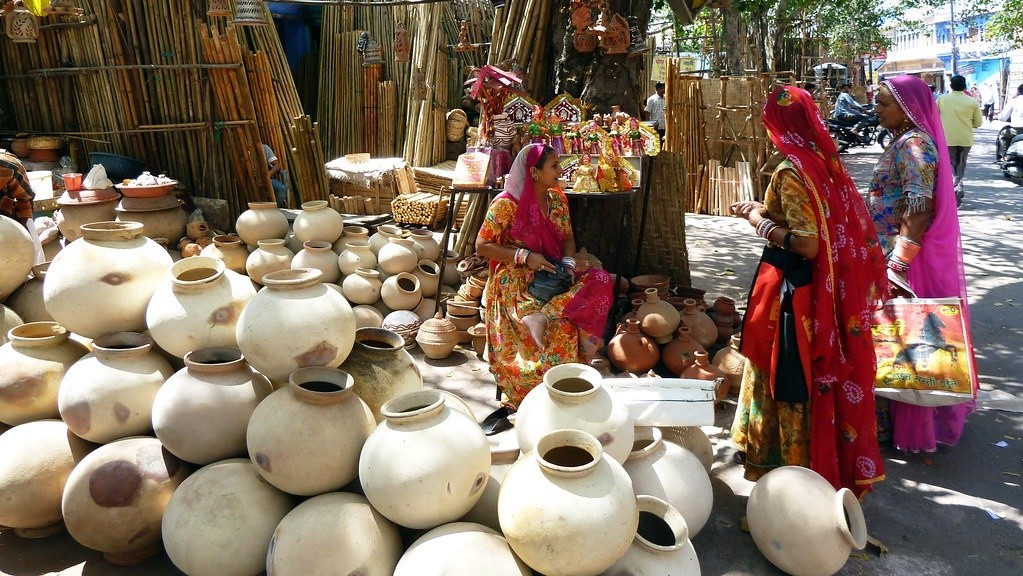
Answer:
[738,233,812,404]
[871,268,978,406]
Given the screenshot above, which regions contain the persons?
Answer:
[527,112,646,193]
[864,79,874,104]
[804,82,816,94]
[866,75,979,455]
[0,149,46,278]
[729,86,885,501]
[475,143,614,410]
[935,75,983,207]
[835,83,873,135]
[927,82,995,123]
[250,144,285,208]
[644,82,666,151]
[997,84,1023,161]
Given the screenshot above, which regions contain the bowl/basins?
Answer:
[27,149,59,162]
[115,179,179,197]
[56,189,121,206]
[88,151,145,179]
[114,196,183,212]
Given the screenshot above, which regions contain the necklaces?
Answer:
[892,124,912,140]
[545,196,551,217]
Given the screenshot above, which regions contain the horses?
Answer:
[883,311,958,377]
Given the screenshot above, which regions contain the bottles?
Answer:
[0,192,869,575]
[11,139,30,157]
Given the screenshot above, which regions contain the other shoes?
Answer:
[850,128,858,134]
[955,190,964,207]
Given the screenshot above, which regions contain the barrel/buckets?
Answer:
[27,170,54,202]
[62,173,82,190]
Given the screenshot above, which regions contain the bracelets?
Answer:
[887,256,909,272]
[560,257,577,269]
[755,218,783,241]
[514,248,531,267]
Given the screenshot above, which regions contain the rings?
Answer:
[538,269,542,271]
[541,265,544,270]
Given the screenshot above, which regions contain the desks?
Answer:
[434,188,633,319]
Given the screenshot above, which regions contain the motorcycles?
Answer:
[993,116,1023,186]
[821,103,894,154]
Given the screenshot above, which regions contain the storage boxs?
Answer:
[558,153,643,189]
